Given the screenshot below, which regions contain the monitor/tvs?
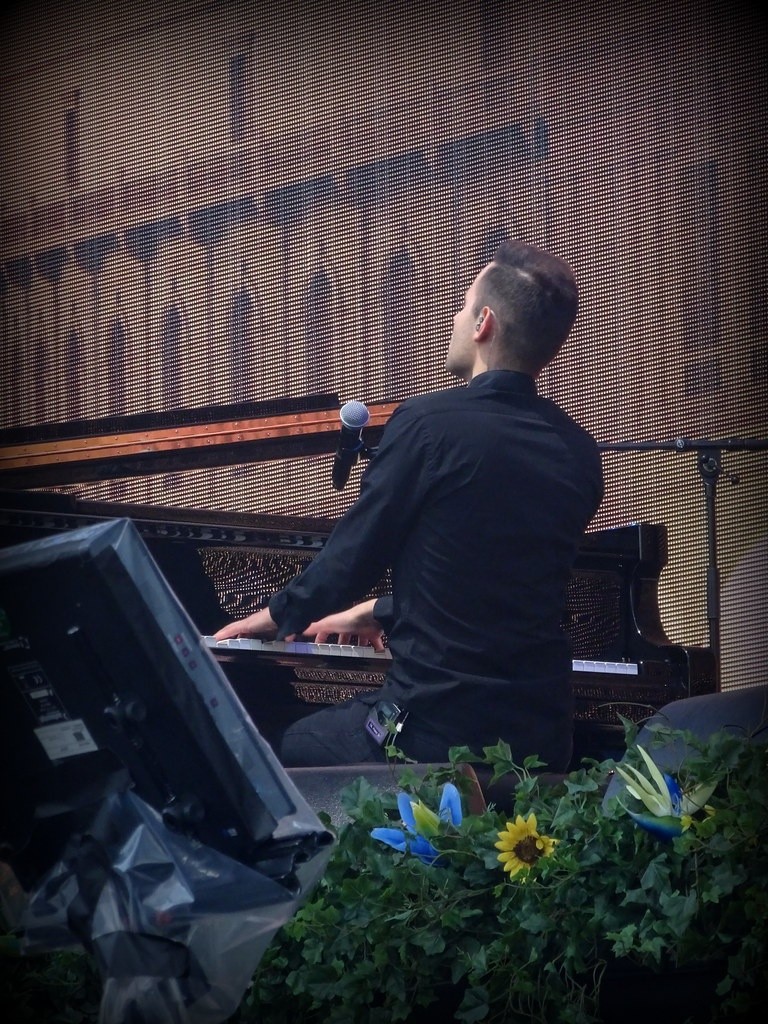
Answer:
[1,515,334,895]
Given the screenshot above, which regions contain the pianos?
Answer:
[0,482,721,731]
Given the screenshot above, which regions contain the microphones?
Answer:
[332,401,370,492]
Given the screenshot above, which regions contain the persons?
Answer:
[218,238,607,764]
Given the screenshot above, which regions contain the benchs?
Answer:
[279,763,618,832]
